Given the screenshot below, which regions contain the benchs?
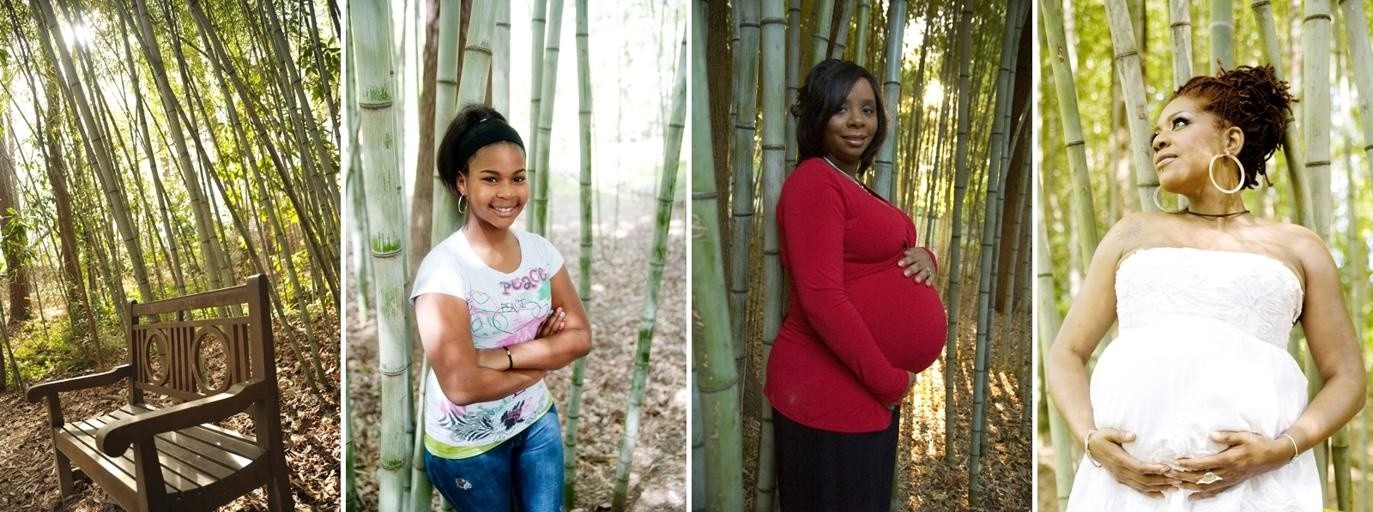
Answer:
[24,273,297,512]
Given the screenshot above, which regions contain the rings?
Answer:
[923,266,932,276]
[1195,469,1222,487]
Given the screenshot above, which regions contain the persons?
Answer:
[406,100,595,512]
[760,55,949,512]
[1041,63,1369,512]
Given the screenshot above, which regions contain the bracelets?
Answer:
[1278,433,1298,464]
[1084,429,1103,469]
[501,346,514,369]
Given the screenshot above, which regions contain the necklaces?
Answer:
[1184,209,1251,218]
